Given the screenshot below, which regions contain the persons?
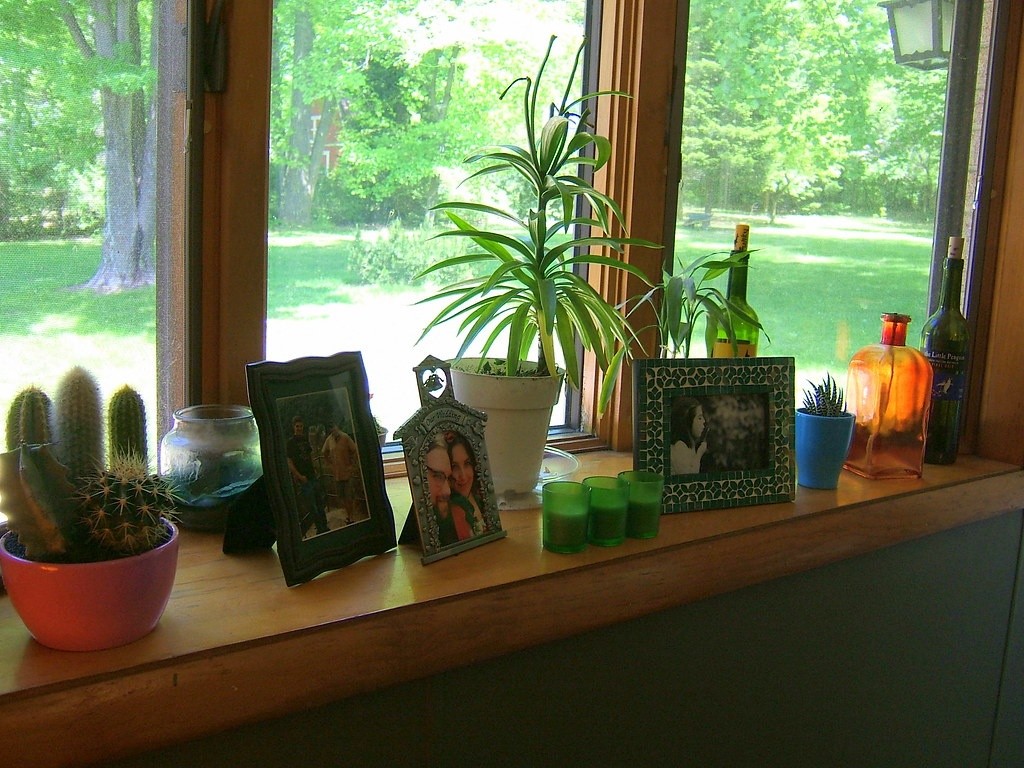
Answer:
[426,432,484,547]
[321,421,357,524]
[286,415,332,535]
[671,397,707,474]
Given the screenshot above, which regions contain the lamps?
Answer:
[876,0,956,70]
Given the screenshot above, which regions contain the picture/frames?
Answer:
[631,356,796,515]
[392,356,509,567]
[244,349,398,588]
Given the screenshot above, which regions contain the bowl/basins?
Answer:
[492,446,580,510]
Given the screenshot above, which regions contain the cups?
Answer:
[582,476,630,547]
[543,481,591,555]
[618,471,664,539]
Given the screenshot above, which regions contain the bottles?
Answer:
[710,223,758,358]
[843,312,933,481]
[919,237,970,466]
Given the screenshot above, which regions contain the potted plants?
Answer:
[794,372,856,489]
[410,33,665,501]
[0,365,183,653]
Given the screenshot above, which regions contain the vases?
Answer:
[844,312,936,480]
[158,404,262,532]
